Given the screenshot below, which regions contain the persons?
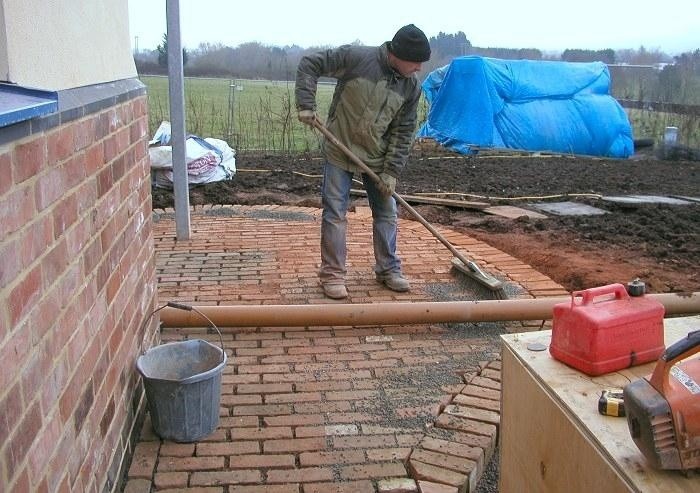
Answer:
[295,25,432,299]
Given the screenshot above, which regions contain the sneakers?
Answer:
[319,276,350,300]
[375,272,412,293]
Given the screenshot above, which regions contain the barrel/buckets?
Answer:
[135,302,225,443]
[663,126,679,142]
[549,282,667,378]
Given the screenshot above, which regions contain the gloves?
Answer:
[375,173,398,199]
[297,110,325,129]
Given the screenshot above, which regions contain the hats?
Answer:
[387,23,432,63]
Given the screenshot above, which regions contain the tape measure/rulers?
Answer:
[598,389,626,417]
